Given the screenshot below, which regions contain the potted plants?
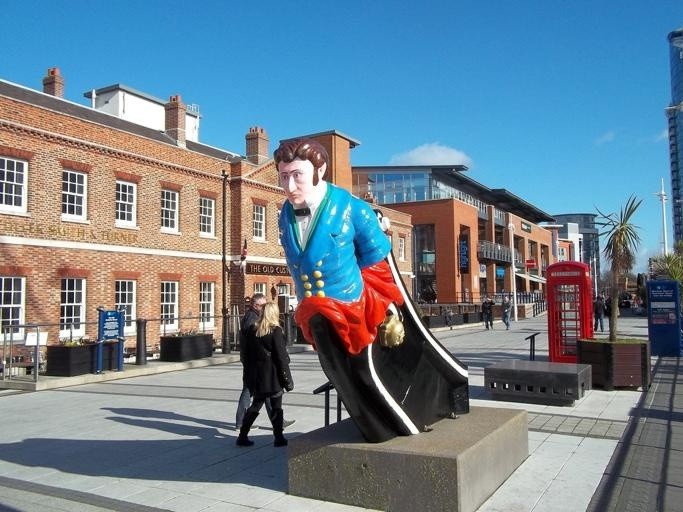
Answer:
[46,336,118,377]
[158,327,213,362]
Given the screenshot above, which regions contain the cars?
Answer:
[620,298,646,317]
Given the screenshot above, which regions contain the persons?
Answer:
[481,297,496,331]
[235,299,295,448]
[272,137,470,446]
[233,291,296,435]
[501,295,513,331]
[592,294,607,332]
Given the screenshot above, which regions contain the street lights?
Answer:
[219,168,231,354]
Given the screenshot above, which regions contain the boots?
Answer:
[271,408,289,447]
[236,411,260,446]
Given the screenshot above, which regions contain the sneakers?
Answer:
[282,417,296,429]
[506,323,514,331]
[487,324,494,330]
[236,425,259,432]
